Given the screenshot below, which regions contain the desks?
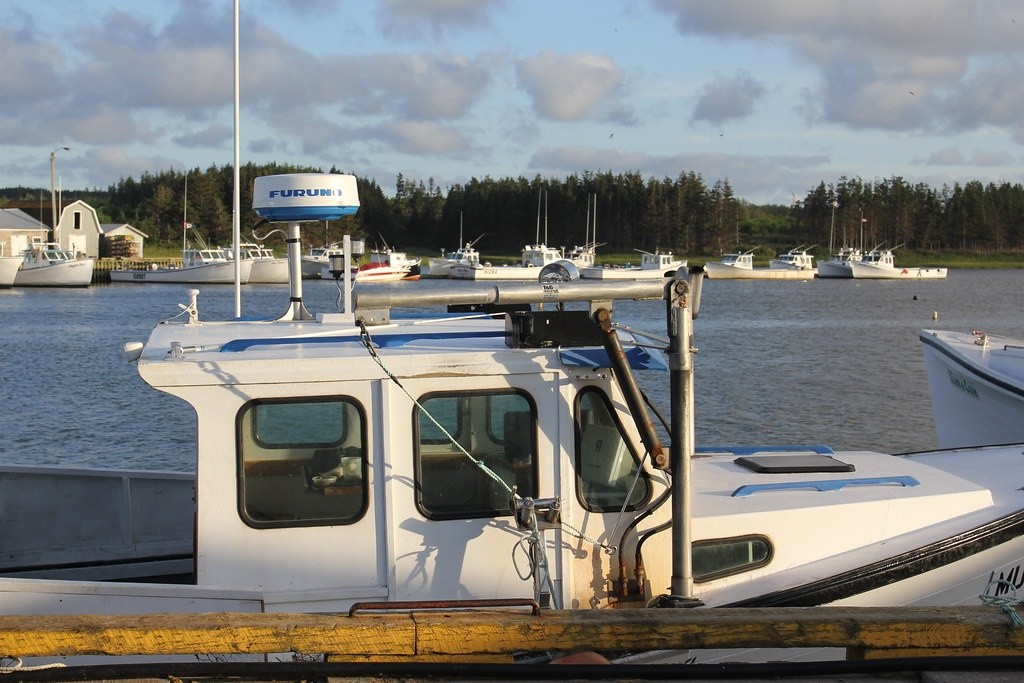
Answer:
[307,486,362,496]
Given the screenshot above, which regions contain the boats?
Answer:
[582,247,688,281]
[109,170,421,286]
[916,327,1023,448]
[0,242,24,289]
[703,243,820,279]
[13,243,94,287]
[851,251,947,279]
[814,247,866,279]
[419,187,608,279]
[0,0,1023,667]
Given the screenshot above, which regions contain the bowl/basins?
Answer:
[312,476,337,488]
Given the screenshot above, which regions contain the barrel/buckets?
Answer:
[341,457,362,482]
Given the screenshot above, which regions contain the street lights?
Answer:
[50,147,70,240]
